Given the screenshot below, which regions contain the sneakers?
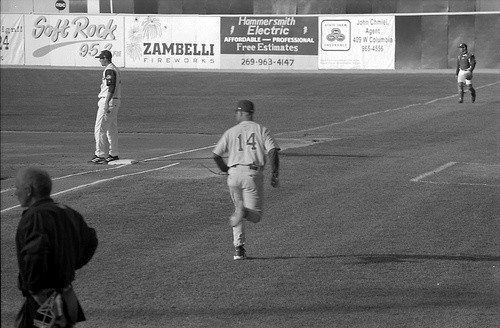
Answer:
[105,155,119,161]
[88,155,105,164]
[230,202,244,227]
[233,246,247,261]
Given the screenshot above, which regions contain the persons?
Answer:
[90,50,121,164]
[13,166,98,328]
[212,99,280,260]
[456,44,476,104]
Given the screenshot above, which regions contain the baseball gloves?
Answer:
[464,70,472,80]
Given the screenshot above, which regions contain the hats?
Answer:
[234,100,254,113]
[458,43,467,48]
[94,50,112,58]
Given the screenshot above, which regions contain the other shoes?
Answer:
[457,98,463,103]
[471,94,476,103]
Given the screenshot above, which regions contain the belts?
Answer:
[231,165,258,170]
[101,97,118,99]
